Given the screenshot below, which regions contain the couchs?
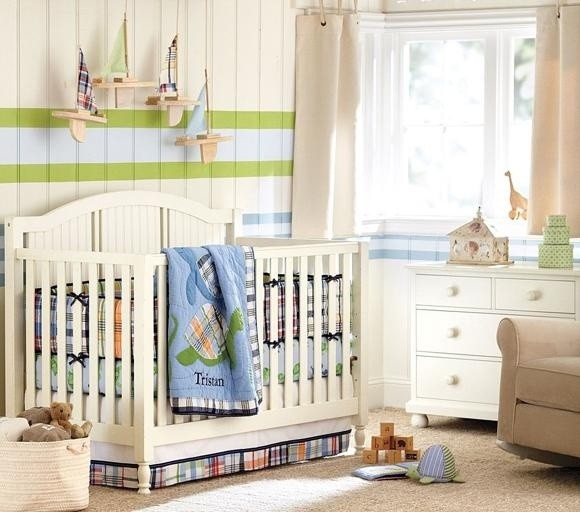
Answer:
[494,317,580,468]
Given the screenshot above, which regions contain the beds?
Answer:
[4,189,366,499]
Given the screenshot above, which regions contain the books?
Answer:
[352,461,420,481]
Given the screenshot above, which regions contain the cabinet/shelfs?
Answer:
[404,259,580,430]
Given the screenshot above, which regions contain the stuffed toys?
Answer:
[49,401,92,439]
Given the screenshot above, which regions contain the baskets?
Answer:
[1,437,91,511]
